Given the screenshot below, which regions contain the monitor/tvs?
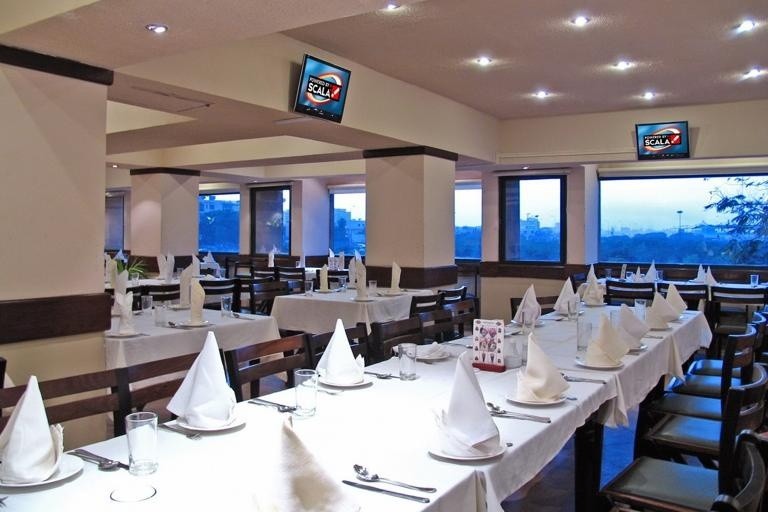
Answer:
[636,121,690,160]
[292,54,351,122]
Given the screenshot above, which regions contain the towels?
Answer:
[320,264,329,292]
[110,262,118,288]
[355,250,362,262]
[633,265,642,282]
[586,263,599,282]
[583,282,605,305]
[300,253,305,268]
[328,248,335,257]
[268,251,275,267]
[619,305,648,346]
[190,280,205,325]
[106,255,112,282]
[112,270,129,311]
[553,277,581,312]
[705,266,716,285]
[667,283,687,313]
[167,254,175,277]
[652,291,677,321]
[165,330,235,429]
[156,255,166,277]
[645,307,668,329]
[179,264,192,306]
[391,261,401,290]
[268,418,361,512]
[193,254,201,276]
[512,284,541,323]
[431,352,500,457]
[0,375,64,484]
[316,319,366,385]
[696,264,706,280]
[356,262,368,297]
[644,261,658,282]
[339,251,344,270]
[116,292,137,334]
[514,334,569,401]
[348,258,355,285]
[585,312,628,367]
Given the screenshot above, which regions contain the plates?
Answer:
[169,304,192,310]
[670,315,683,320]
[315,289,333,293]
[0,452,84,487]
[558,310,584,315]
[581,301,608,306]
[311,376,373,388]
[156,276,167,280]
[515,320,545,326]
[105,330,139,337]
[429,433,505,461]
[111,310,141,316]
[689,279,703,283]
[649,325,672,330]
[351,295,375,302]
[416,345,450,362]
[343,284,359,290]
[160,281,179,286]
[178,320,209,327]
[505,388,568,405]
[175,409,244,432]
[384,290,406,296]
[629,344,647,351]
[575,356,625,368]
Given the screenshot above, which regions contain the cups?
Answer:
[339,276,346,291]
[656,270,664,282]
[219,269,226,279]
[605,268,613,280]
[398,343,417,381]
[626,272,633,284]
[305,280,314,298]
[751,274,760,287]
[140,296,151,318]
[576,318,592,349]
[132,273,139,284]
[295,368,316,415]
[125,412,159,474]
[368,279,378,297]
[177,268,183,281]
[635,299,646,318]
[296,261,299,268]
[222,295,232,317]
[155,304,166,324]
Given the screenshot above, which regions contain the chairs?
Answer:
[225,257,251,311]
[436,299,478,340]
[687,313,766,379]
[597,363,768,512]
[105,285,145,311]
[370,315,426,359]
[643,324,758,471]
[307,322,367,367]
[199,279,241,313]
[606,280,655,304]
[121,345,227,435]
[438,286,467,338]
[510,295,559,324]
[710,428,768,512]
[569,272,587,294]
[316,270,349,289]
[657,283,708,309]
[667,374,741,399]
[1,368,122,441]
[250,266,277,315]
[414,309,452,344]
[224,333,307,403]
[409,292,446,319]
[648,392,722,421]
[278,268,305,296]
[144,284,180,309]
[711,286,768,360]
[249,281,289,314]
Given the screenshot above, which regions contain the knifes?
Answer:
[365,370,399,378]
[567,375,606,384]
[490,412,551,424]
[252,397,298,411]
[76,448,129,470]
[68,446,127,471]
[342,479,430,503]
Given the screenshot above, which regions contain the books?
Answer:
[327,257,340,272]
[472,319,506,372]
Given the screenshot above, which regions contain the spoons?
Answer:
[540,318,564,322]
[402,288,420,292]
[561,372,604,383]
[67,452,119,470]
[166,321,190,330]
[506,329,522,335]
[248,401,291,413]
[486,402,550,420]
[168,321,189,330]
[642,334,663,339]
[233,312,254,321]
[446,342,472,348]
[365,372,391,378]
[353,464,436,493]
